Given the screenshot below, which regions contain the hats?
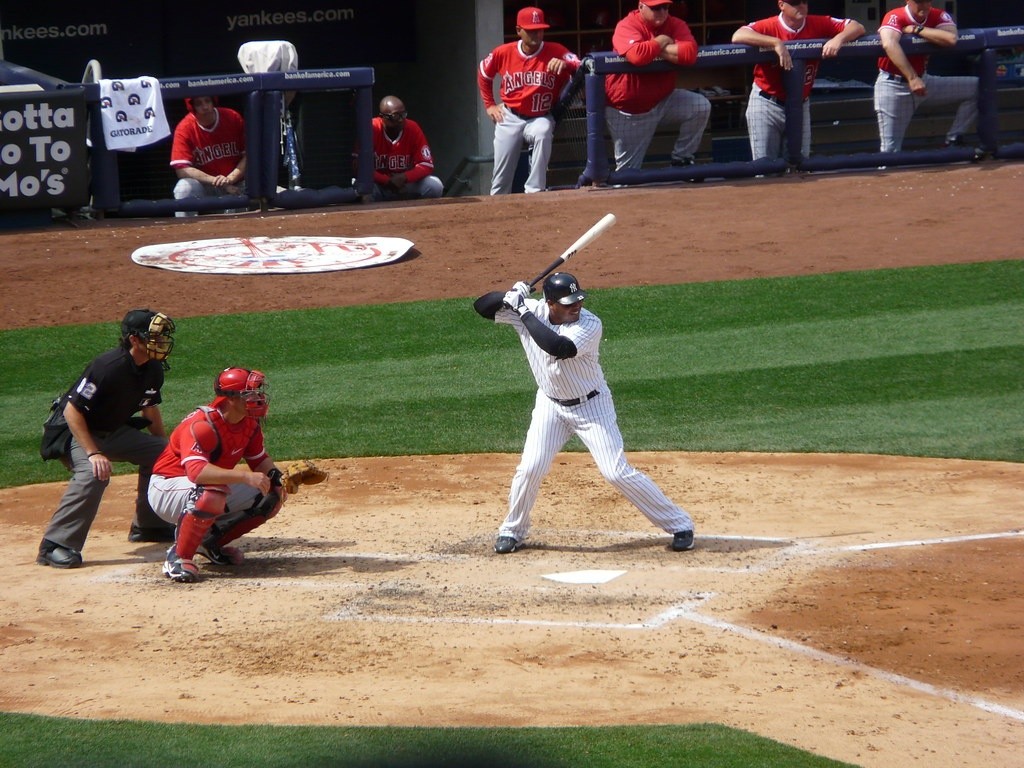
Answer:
[121,309,173,337]
[640,0,673,7]
[517,7,550,30]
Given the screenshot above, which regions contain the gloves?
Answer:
[503,289,529,317]
[510,280,536,299]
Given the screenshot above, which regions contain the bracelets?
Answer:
[912,25,924,35]
[87,450,104,458]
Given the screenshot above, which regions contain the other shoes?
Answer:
[943,134,966,147]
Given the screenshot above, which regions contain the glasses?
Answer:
[379,112,407,122]
[647,5,669,10]
[788,0,807,6]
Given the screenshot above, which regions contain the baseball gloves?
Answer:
[279,460,328,495]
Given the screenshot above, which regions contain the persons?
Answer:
[873,0,980,170]
[148,368,298,583]
[34,310,176,569]
[474,272,695,554]
[351,96,443,204]
[170,96,246,217]
[477,7,581,196]
[731,0,867,177]
[603,0,712,189]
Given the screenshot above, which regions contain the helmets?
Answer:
[543,272,587,305]
[210,368,251,409]
[184,94,218,115]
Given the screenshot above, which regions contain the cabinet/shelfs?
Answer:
[503,0,750,61]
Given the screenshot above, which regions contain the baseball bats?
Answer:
[502,213,616,309]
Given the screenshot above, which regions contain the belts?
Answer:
[760,91,809,107]
[888,72,922,83]
[546,389,600,406]
[504,104,548,120]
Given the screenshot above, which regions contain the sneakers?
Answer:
[672,529,694,551]
[495,535,522,553]
[162,545,193,583]
[669,158,704,183]
[194,540,229,565]
[128,523,175,542]
[35,538,80,567]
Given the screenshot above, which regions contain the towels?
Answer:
[99,75,172,154]
